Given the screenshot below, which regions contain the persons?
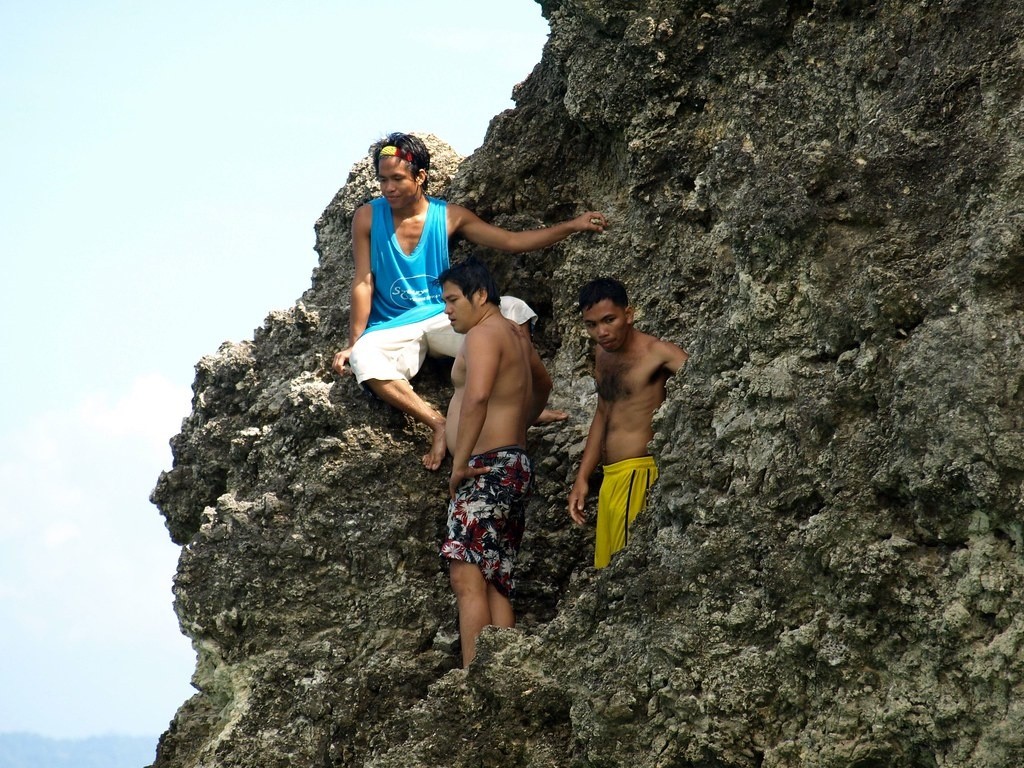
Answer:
[569,277,689,568]
[334,132,606,470]
[438,257,553,668]
